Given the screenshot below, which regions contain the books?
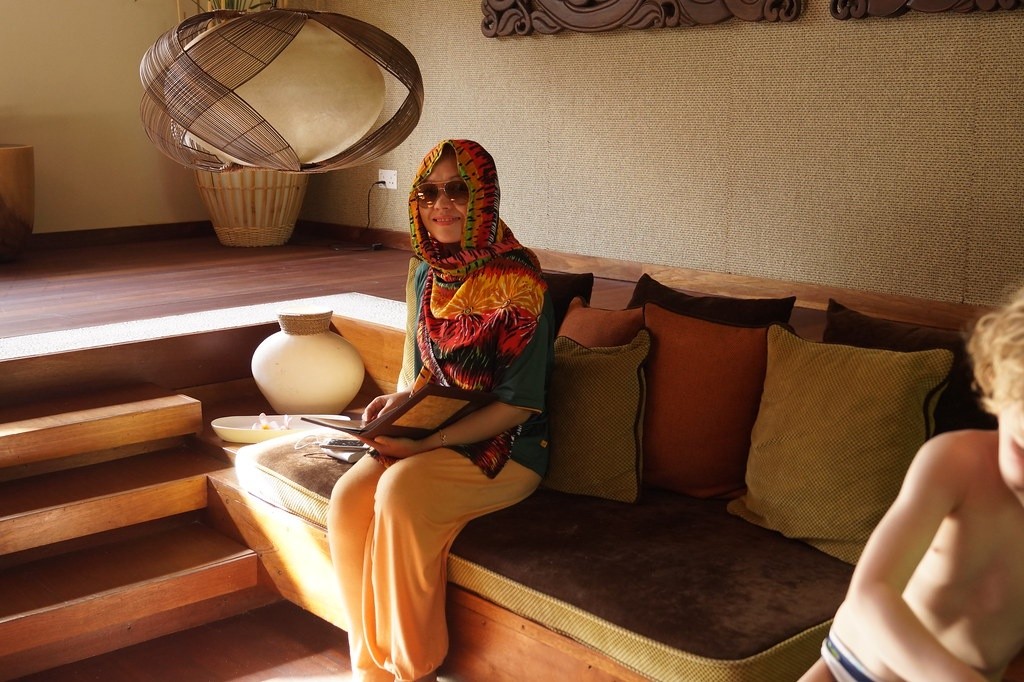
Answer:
[301,383,500,440]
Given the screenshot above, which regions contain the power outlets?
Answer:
[379,169,398,190]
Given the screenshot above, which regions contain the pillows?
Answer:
[638,299,796,505]
[539,270,594,342]
[540,328,656,511]
[727,319,961,562]
[555,295,645,349]
[622,269,797,340]
[823,298,1001,438]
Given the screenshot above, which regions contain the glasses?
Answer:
[408,180,470,209]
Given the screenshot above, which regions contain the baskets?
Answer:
[193,167,309,246]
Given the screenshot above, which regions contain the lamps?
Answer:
[139,0,424,177]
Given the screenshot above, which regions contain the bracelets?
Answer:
[439,430,448,448]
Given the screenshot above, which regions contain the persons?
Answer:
[795,288,1024,681]
[324,137,553,681]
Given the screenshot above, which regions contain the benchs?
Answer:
[171,262,1024,682]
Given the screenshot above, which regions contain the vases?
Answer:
[251,309,364,415]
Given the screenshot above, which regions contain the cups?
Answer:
[0,144,34,263]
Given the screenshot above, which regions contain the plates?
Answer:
[211,415,351,442]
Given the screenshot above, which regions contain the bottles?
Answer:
[251,307,365,414]
